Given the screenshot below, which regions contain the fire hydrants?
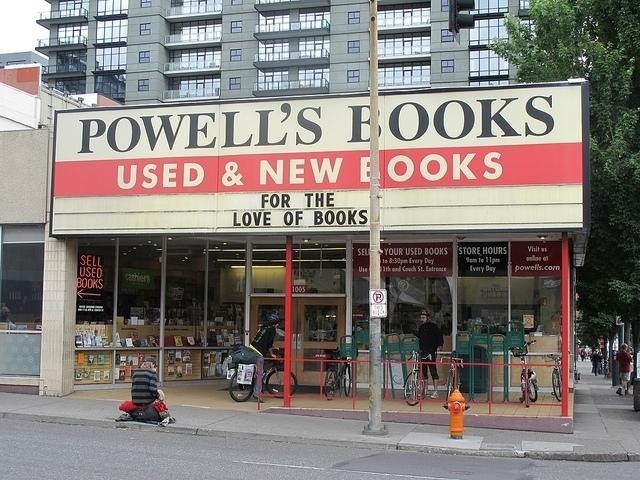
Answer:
[443,390,472,440]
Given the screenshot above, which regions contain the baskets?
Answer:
[509,342,528,357]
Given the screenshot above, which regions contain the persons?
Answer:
[413,311,444,398]
[132,357,167,407]
[580,342,632,396]
[249,311,279,402]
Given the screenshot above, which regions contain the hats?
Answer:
[144,358,157,368]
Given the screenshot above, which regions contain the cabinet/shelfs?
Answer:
[339,335,562,386]
[74,325,243,384]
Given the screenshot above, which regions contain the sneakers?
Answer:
[253,394,266,403]
[431,391,439,399]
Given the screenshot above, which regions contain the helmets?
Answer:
[528,371,536,382]
[267,314,280,326]
[420,310,431,316]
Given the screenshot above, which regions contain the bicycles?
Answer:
[509,339,539,404]
[402,351,433,406]
[441,353,464,404]
[549,353,563,402]
[323,349,352,398]
[229,346,298,402]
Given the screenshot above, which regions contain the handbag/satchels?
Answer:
[230,345,259,364]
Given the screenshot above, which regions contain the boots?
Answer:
[616,388,628,395]
